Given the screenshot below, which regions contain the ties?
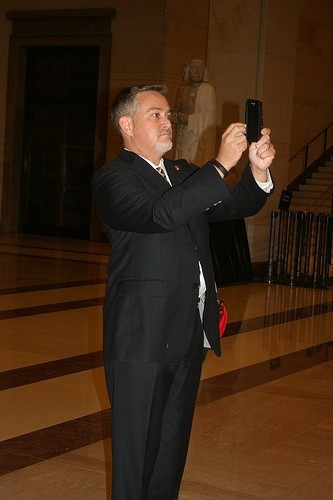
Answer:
[157,167,168,180]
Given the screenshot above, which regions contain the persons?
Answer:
[171,60,217,168]
[88,85,275,500]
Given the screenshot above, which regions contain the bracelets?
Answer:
[210,158,228,177]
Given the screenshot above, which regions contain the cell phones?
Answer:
[244,99,260,142]
